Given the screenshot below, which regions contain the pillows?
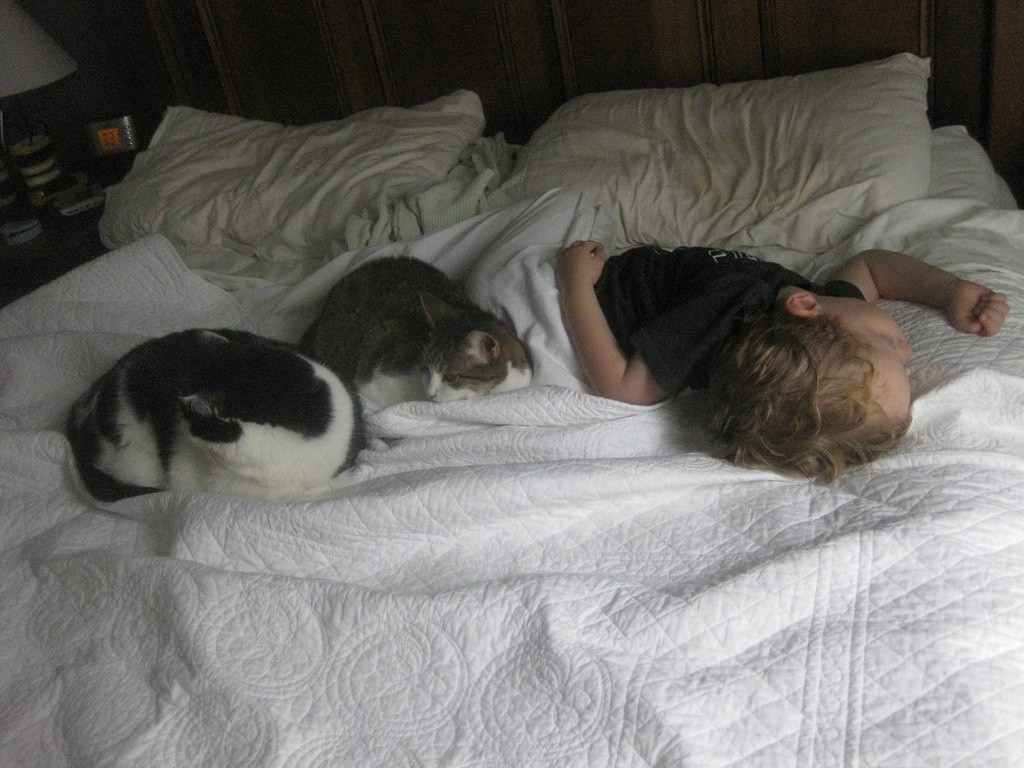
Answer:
[490,58,933,255]
[98,89,485,261]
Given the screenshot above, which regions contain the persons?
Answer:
[555,238,1011,484]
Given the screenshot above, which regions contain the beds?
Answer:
[1,0,1024,768]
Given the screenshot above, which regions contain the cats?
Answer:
[295,254,534,403]
[61,326,366,503]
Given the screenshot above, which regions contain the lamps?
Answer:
[0,0,79,191]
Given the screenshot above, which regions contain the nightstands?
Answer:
[0,160,124,302]
